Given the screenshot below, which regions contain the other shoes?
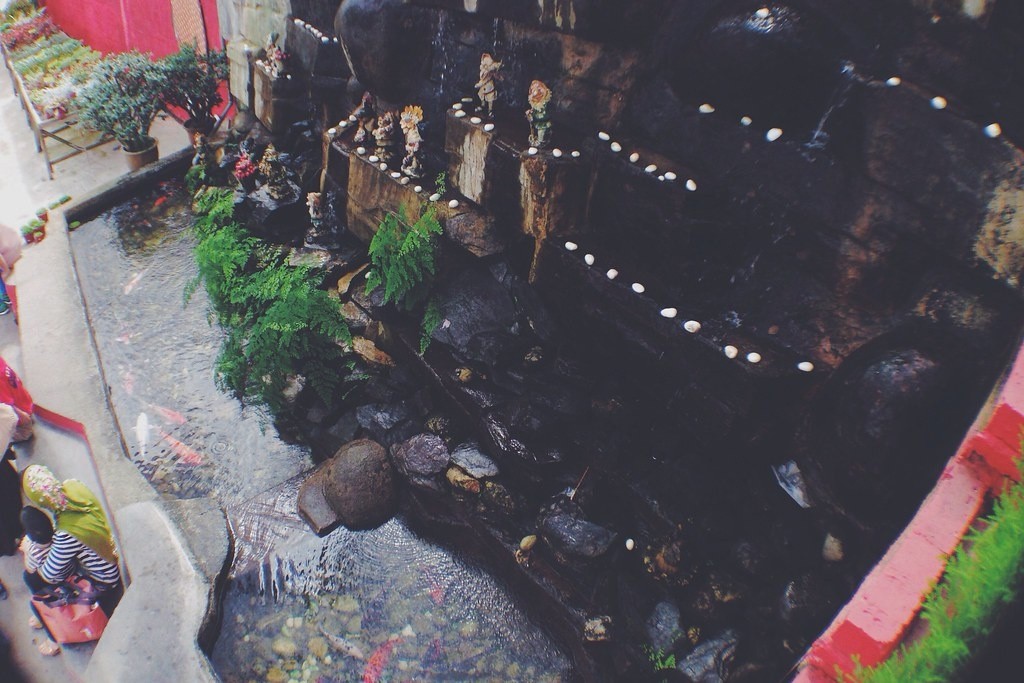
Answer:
[29,616,61,655]
[0,293,16,316]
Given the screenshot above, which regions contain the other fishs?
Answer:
[114,179,204,471]
[319,584,445,681]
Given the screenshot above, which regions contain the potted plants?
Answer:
[158,36,230,144]
[72,49,168,170]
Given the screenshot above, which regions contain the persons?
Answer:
[0,358,36,445]
[16,463,123,655]
[1,403,28,598]
[0,224,20,316]
[18,505,75,586]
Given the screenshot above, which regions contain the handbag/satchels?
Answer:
[32,576,109,646]
